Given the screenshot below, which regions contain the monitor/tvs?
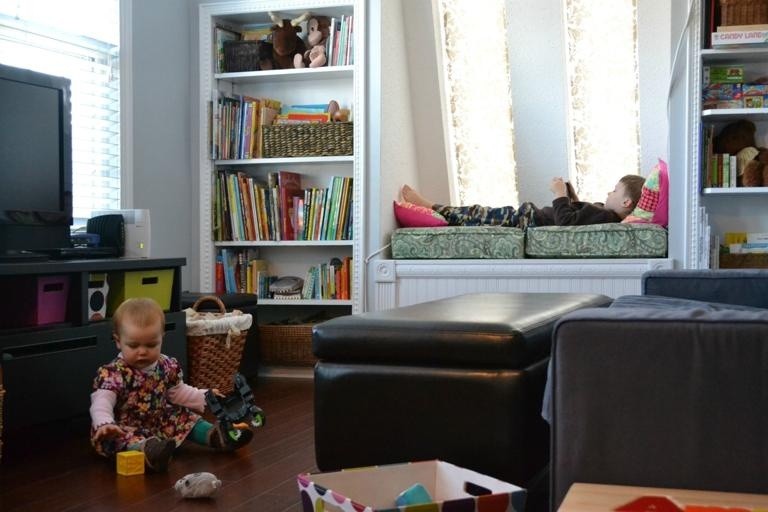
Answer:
[0,64,74,226]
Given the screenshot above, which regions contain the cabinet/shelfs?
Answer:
[0,253,189,469]
[694,0,767,271]
[198,0,416,382]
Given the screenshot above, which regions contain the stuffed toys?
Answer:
[174,470,223,498]
[260,12,308,70]
[304,16,330,68]
[722,119,767,187]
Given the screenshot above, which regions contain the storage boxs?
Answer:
[25,271,71,329]
[109,269,175,312]
[292,455,534,512]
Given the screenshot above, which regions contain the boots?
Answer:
[142,436,177,478]
[208,421,254,453]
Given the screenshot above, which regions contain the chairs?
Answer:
[541,260,766,512]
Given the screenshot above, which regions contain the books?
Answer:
[717,24,767,34]
[711,233,721,268]
[328,14,355,66]
[214,26,239,74]
[701,123,736,187]
[701,207,710,267]
[711,33,768,46]
[211,88,352,298]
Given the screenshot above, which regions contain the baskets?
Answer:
[177,293,253,394]
[256,325,319,367]
[260,123,352,158]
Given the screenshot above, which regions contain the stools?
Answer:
[309,288,612,510]
[181,290,259,384]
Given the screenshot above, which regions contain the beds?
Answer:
[365,220,683,313]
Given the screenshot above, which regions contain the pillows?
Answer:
[619,154,669,225]
[390,197,448,229]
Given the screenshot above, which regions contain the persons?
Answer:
[399,174,647,226]
[89,297,254,471]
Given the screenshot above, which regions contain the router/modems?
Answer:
[87,215,125,257]
